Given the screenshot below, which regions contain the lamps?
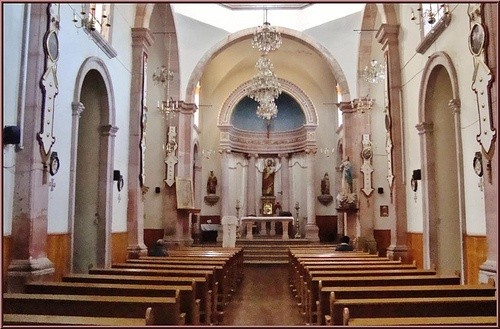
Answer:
[155,65,174,87]
[246,8,281,119]
[362,58,385,85]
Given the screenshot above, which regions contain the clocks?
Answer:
[50,153,60,176]
[117,178,124,192]
[411,178,417,192]
[470,153,483,178]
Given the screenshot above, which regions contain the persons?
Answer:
[148,239,166,257]
[335,236,353,251]
[321,172,330,195]
[207,170,217,194]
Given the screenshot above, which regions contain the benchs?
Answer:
[287,246,498,328]
[1,245,244,326]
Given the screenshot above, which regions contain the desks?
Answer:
[241,215,294,242]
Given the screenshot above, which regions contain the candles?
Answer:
[157,100,160,108]
[167,100,169,108]
[172,103,175,110]
[176,101,178,109]
[162,104,164,111]
